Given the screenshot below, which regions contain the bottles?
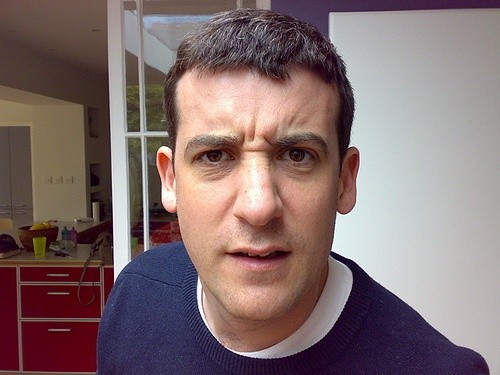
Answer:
[61,227,77,248]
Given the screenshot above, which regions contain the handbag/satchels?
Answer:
[77,232,113,305]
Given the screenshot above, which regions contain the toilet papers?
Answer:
[92,201,101,223]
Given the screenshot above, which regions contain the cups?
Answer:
[32,236,46,258]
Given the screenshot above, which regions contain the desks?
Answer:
[0,216,112,242]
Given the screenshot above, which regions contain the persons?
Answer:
[97,7,490,375]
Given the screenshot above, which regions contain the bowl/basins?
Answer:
[18,225,59,251]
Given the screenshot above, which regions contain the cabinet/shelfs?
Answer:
[86,104,110,217]
[0,244,114,375]
